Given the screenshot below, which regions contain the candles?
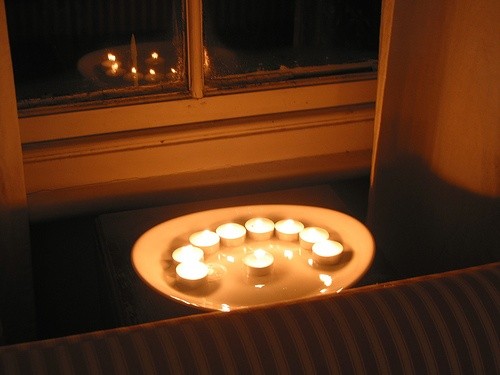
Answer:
[99,43,211,84]
[170,215,346,291]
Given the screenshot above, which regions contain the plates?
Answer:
[76,41,243,92]
[129,204,375,312]
